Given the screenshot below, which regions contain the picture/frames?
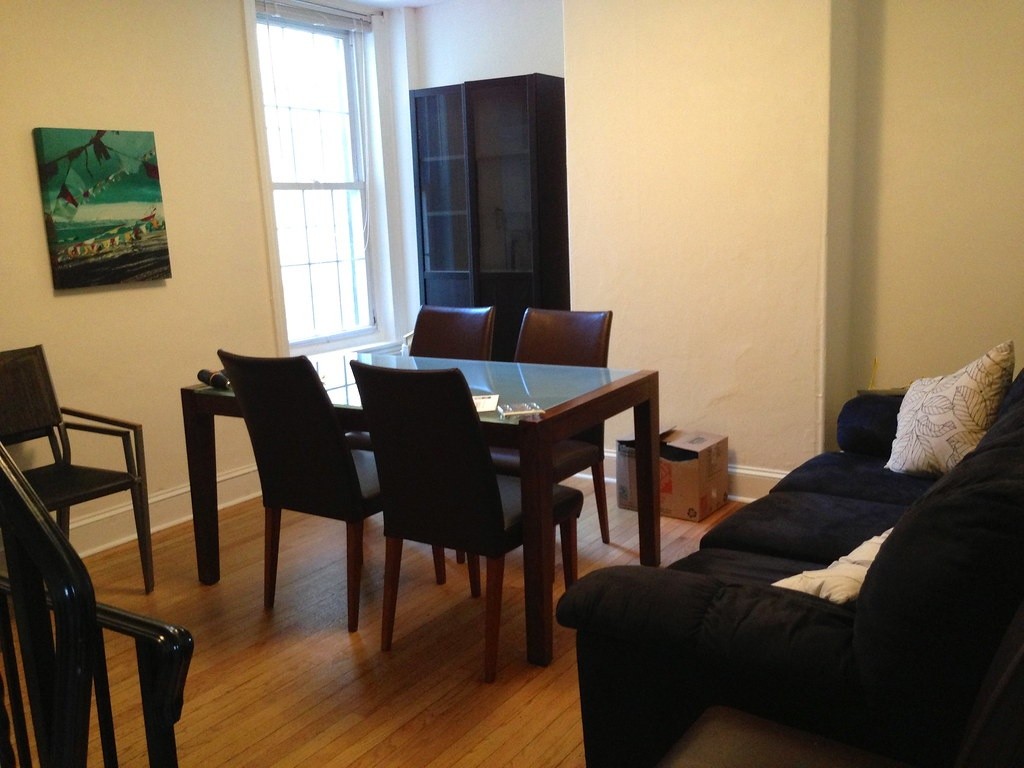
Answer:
[32,126,172,290]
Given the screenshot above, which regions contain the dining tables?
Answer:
[180,350,662,667]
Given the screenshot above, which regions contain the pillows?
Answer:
[883,339,1016,482]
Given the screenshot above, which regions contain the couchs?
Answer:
[554,368,1024,768]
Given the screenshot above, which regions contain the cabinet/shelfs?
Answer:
[410,72,571,365]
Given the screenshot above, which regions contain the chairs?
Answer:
[0,345,156,595]
[514,307,613,545]
[651,598,1024,768]
[216,349,447,635]
[408,304,499,565]
[350,359,585,685]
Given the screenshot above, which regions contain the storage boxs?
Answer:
[615,425,728,523]
